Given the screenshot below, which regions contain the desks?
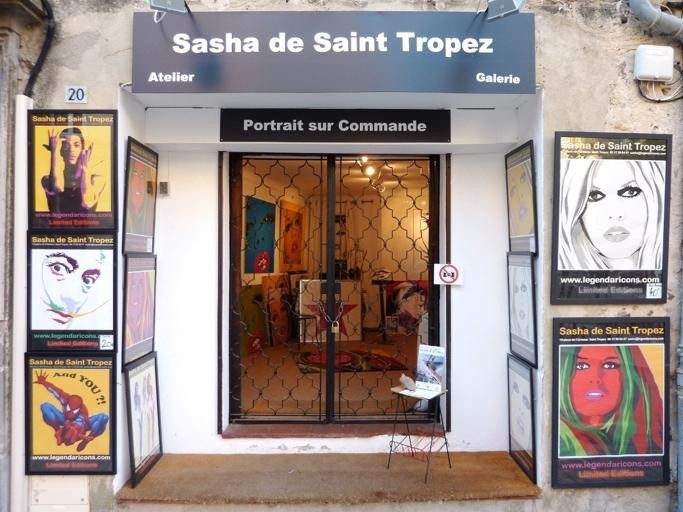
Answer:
[384,382,452,484]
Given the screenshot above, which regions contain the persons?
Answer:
[508,367,531,453]
[560,159,666,271]
[506,162,534,235]
[390,285,426,329]
[40,127,105,213]
[510,266,533,342]
[31,250,105,329]
[127,156,152,239]
[560,347,666,455]
[126,272,155,343]
[33,369,109,452]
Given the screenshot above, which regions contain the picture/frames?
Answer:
[550,317,671,490]
[21,109,120,473]
[548,130,671,306]
[503,138,539,487]
[119,134,164,488]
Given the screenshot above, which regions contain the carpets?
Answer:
[290,348,408,375]
